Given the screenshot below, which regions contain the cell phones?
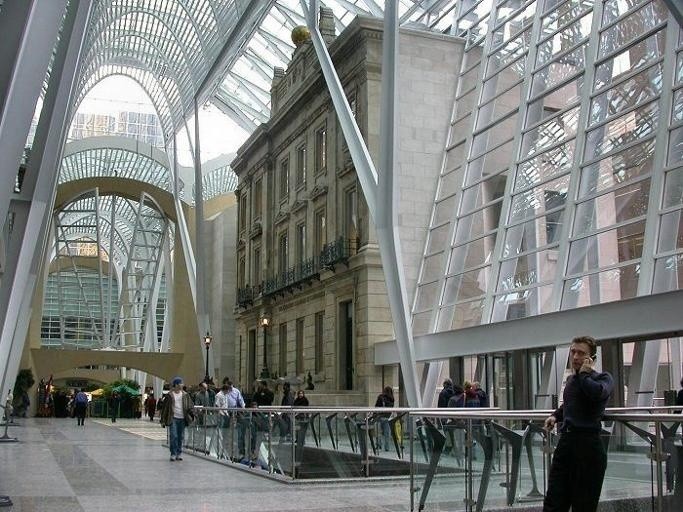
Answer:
[591,354,596,362]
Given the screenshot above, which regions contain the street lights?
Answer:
[259,311,271,378]
[203,330,213,385]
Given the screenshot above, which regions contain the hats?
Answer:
[171,377,182,387]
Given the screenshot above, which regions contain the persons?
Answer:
[109,390,119,422]
[373,386,393,451]
[143,376,315,461]
[435,377,490,459]
[543,335,615,511]
[22,390,30,417]
[47,388,88,425]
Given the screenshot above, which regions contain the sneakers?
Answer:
[170,454,183,461]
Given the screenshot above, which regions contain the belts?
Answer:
[562,426,595,434]
